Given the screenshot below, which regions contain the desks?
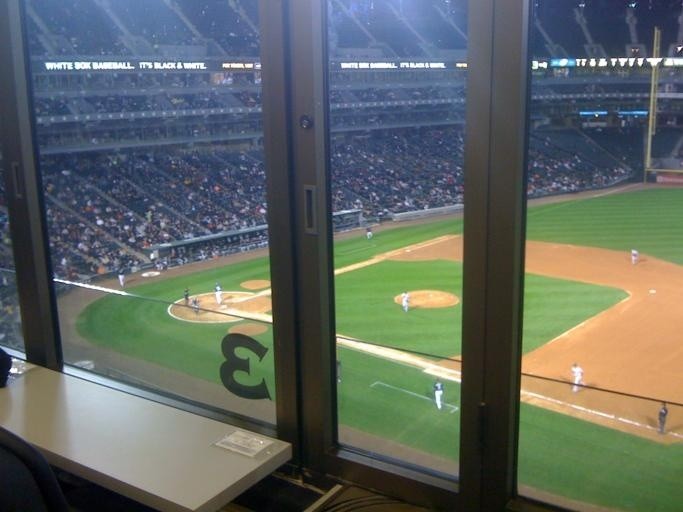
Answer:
[0,354,293,512]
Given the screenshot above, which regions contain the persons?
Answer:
[433,377,443,411]
[630,247,638,266]
[401,289,408,312]
[570,362,585,393]
[657,402,667,435]
[364,223,372,239]
[1,232,276,355]
[1,0,682,231]
